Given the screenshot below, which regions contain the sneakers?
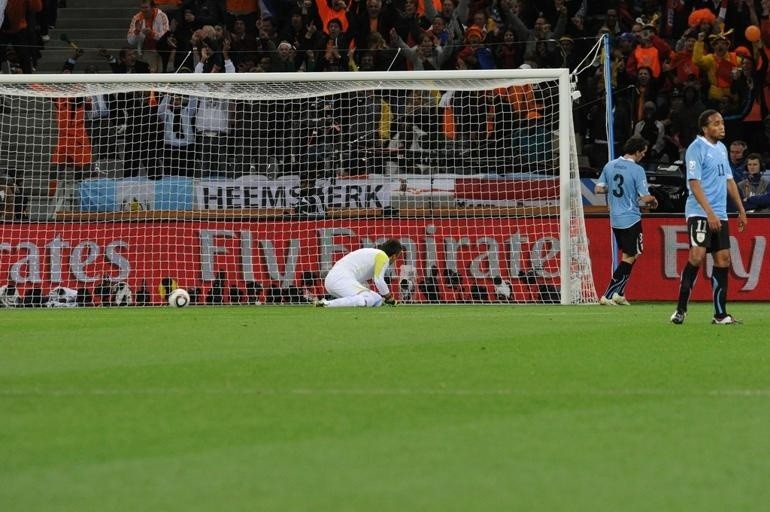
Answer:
[670,310,685,324]
[712,315,736,324]
[315,298,327,307]
[601,292,631,306]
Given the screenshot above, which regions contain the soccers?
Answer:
[168,289,191,310]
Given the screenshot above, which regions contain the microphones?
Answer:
[736,158,746,161]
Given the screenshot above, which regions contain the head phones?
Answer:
[728,141,749,157]
[744,154,766,172]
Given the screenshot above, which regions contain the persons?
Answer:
[670,110,747,324]
[314,238,402,307]
[595,136,658,305]
[1,1,770,213]
